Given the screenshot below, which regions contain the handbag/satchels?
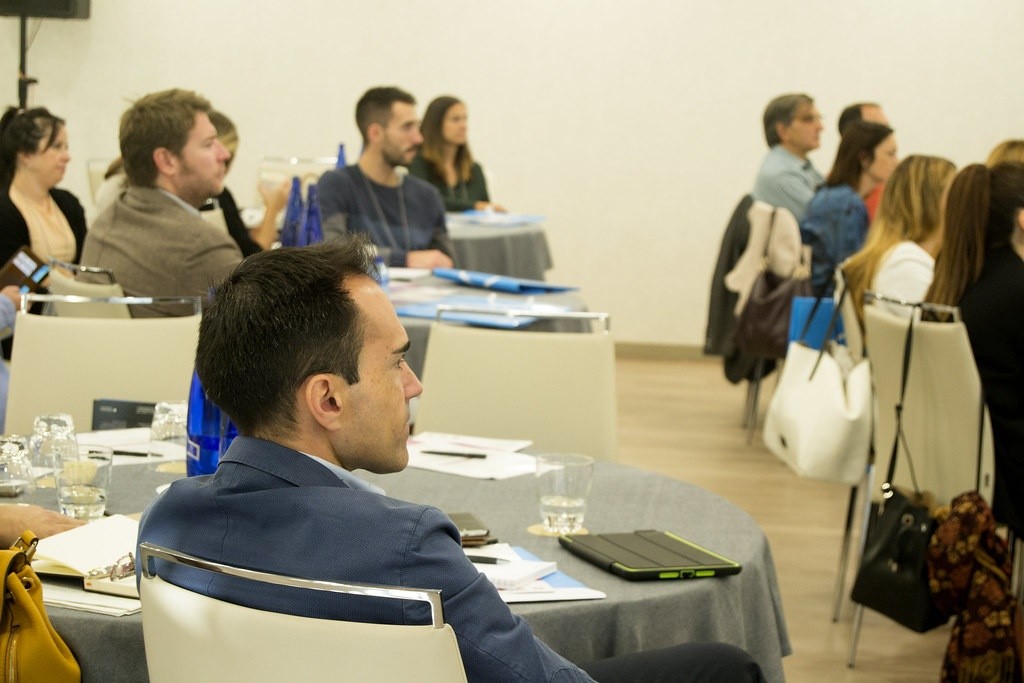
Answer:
[762,339,872,485]
[0,530,81,683]
[848,486,957,634]
[727,209,814,360]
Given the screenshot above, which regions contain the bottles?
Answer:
[281,175,304,248]
[336,144,346,169]
[373,255,389,293]
[185,368,239,478]
[297,184,324,247]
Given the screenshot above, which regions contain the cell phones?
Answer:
[446,511,489,538]
[21,265,50,295]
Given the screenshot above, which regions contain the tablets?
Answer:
[559,529,741,583]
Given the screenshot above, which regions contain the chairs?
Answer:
[701,194,1000,668]
[5,290,204,442]
[420,306,615,448]
[45,255,136,320]
[386,267,599,374]
[134,541,467,682]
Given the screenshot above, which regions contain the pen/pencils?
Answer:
[112,449,164,460]
[422,448,487,460]
[467,556,511,565]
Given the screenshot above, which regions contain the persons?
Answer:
[75,87,247,320]
[0,503,144,551]
[798,119,900,299]
[0,107,88,360]
[750,94,827,225]
[410,95,511,215]
[314,86,456,269]
[987,140,1024,170]
[837,102,889,226]
[133,232,768,683]
[101,111,263,262]
[0,285,34,434]
[839,154,957,356]
[920,160,1024,544]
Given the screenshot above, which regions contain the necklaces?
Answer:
[362,172,411,254]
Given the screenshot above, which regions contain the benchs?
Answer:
[213,204,557,278]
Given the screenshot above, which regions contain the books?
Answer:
[463,547,558,589]
[445,512,491,547]
[32,511,141,601]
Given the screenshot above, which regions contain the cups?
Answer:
[0,412,79,504]
[146,402,188,474]
[535,452,595,535]
[53,444,115,524]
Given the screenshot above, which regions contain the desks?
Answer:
[1,424,796,683]
